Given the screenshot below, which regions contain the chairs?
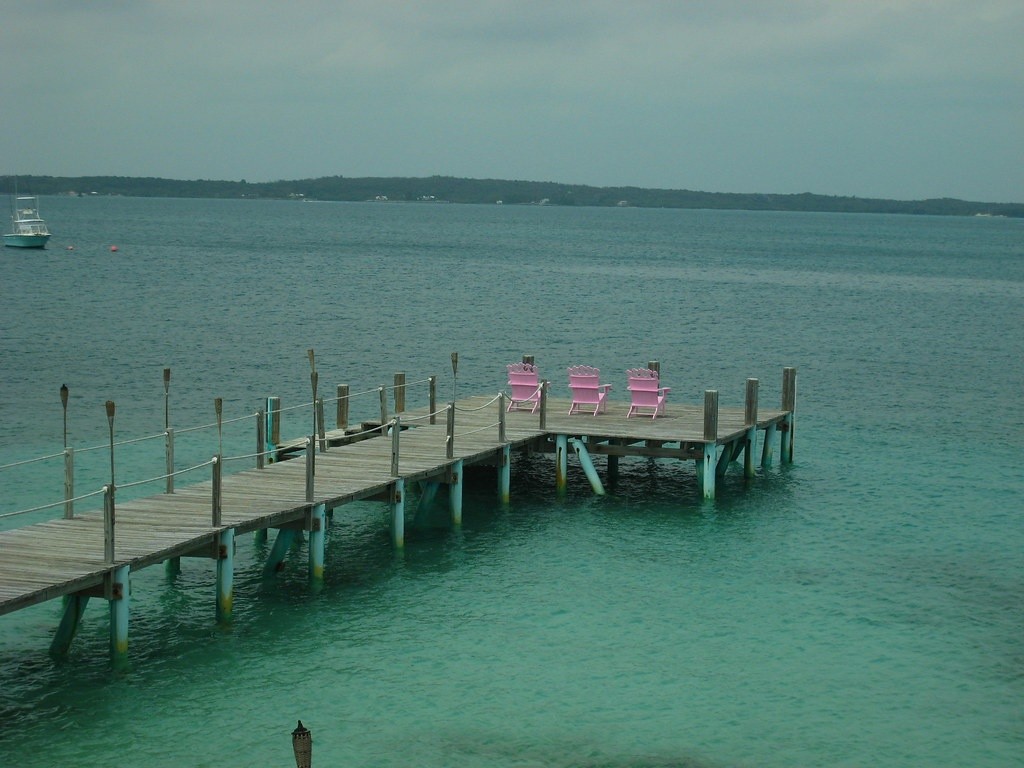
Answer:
[505,362,549,414]
[567,365,612,416]
[626,367,672,419]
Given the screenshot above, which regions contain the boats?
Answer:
[0,180,52,248]
